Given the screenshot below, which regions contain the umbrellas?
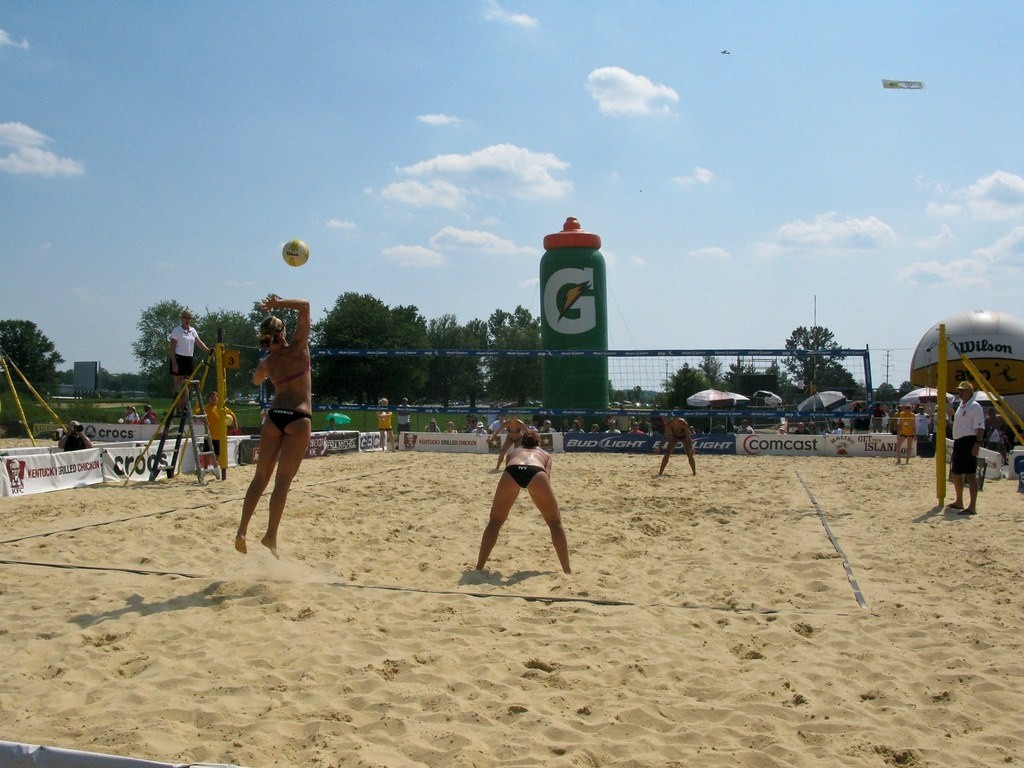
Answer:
[900,385,1005,414]
[753,389,782,406]
[326,413,351,424]
[798,391,853,433]
[686,389,749,433]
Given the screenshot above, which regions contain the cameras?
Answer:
[73,425,84,433]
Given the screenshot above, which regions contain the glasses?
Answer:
[279,321,286,334]
[183,317,191,320]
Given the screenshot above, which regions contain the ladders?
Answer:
[148,378,222,484]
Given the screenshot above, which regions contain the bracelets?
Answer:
[975,440,982,446]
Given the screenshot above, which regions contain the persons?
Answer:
[323,419,335,430]
[395,397,411,450]
[946,381,985,514]
[235,295,312,559]
[195,390,238,470]
[657,411,696,475]
[261,408,270,426]
[848,401,1024,467]
[489,414,532,469]
[794,417,845,436]
[52,420,94,452]
[377,399,396,453]
[423,419,441,433]
[444,422,459,434]
[778,425,787,434]
[460,403,754,435]
[124,403,173,425]
[169,309,211,415]
[475,430,572,574]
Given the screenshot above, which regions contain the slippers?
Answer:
[957,509,977,515]
[946,504,964,509]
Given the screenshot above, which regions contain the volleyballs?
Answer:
[283,238,310,265]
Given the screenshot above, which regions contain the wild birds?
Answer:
[721,49,730,55]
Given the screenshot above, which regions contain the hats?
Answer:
[956,381,973,391]
[779,426,786,431]
[935,405,938,410]
[919,405,924,408]
[476,422,483,428]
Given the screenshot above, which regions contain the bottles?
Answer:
[540,216,609,412]
